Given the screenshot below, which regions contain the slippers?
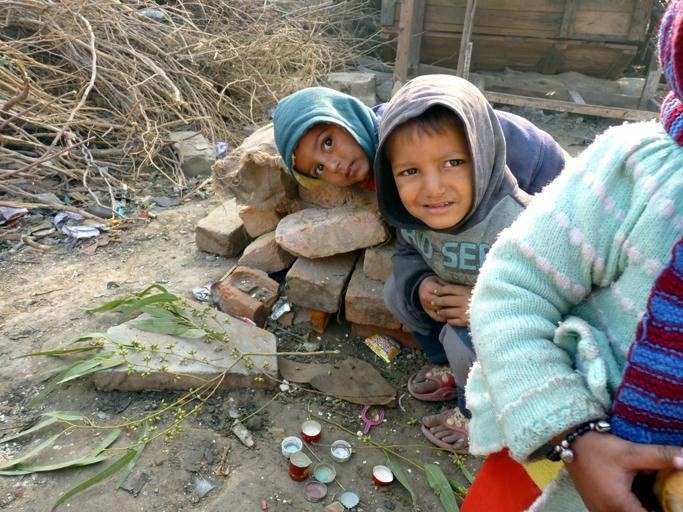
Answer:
[408,364,457,403]
[421,408,468,455]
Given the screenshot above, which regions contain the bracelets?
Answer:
[547,420,610,463]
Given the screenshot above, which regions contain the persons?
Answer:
[274,86,573,198]
[460,1,683,512]
[375,73,537,450]
